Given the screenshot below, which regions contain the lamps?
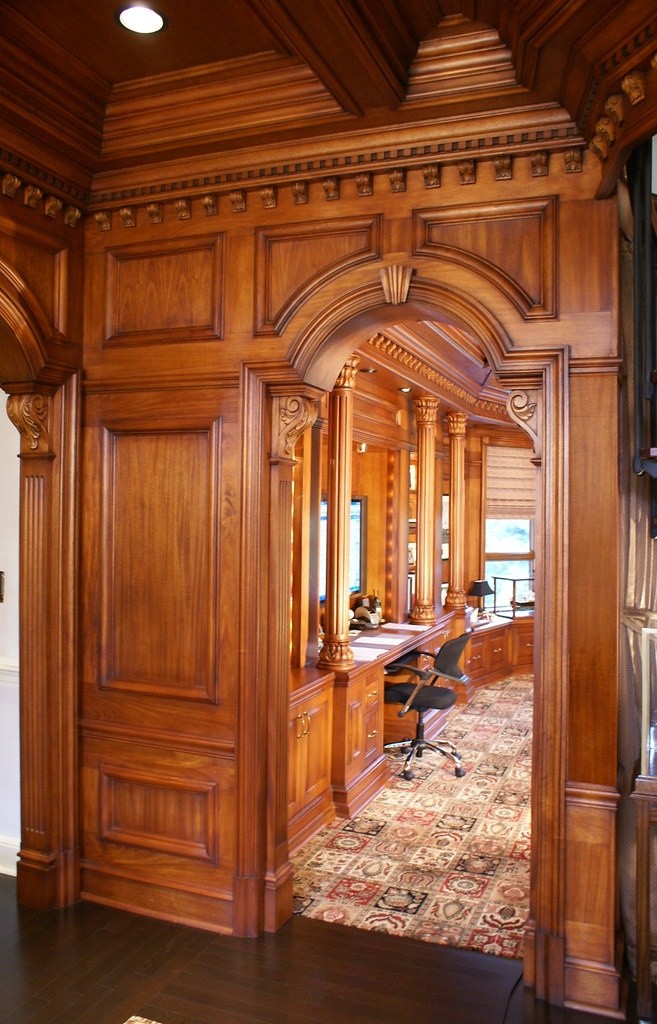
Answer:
[467,580,495,618]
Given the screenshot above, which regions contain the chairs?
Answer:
[384,627,472,781]
